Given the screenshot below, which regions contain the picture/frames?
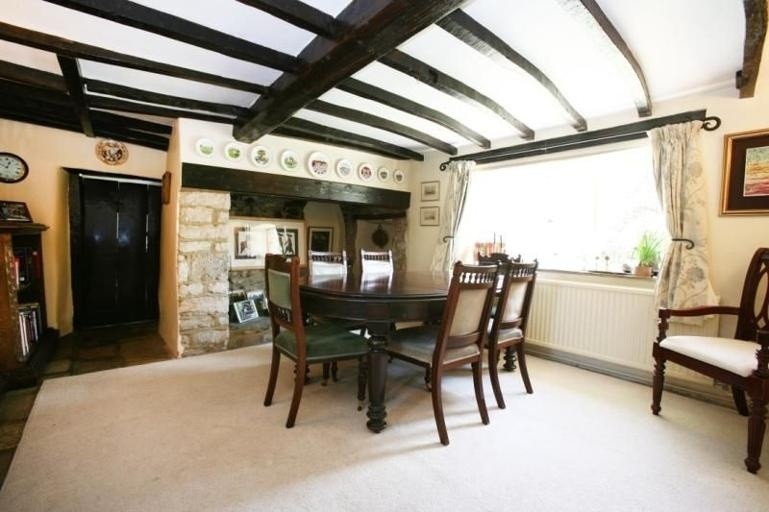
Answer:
[420,206,439,226]
[233,300,259,324]
[308,226,334,251]
[233,227,256,259]
[421,181,440,201]
[718,128,769,217]
[0,200,33,223]
[277,228,298,256]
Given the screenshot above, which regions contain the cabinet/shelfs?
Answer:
[0,221,59,391]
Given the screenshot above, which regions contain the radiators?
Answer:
[524,278,721,388]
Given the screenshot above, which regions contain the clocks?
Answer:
[0,152,29,183]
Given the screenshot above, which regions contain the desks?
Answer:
[297,271,519,434]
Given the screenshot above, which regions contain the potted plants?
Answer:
[632,231,665,277]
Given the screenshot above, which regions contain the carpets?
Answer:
[0,342,769,512]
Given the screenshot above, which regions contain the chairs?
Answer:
[359,249,397,362]
[306,249,348,381]
[650,247,769,474]
[369,259,502,446]
[264,253,372,428]
[477,251,522,367]
[484,259,539,409]
